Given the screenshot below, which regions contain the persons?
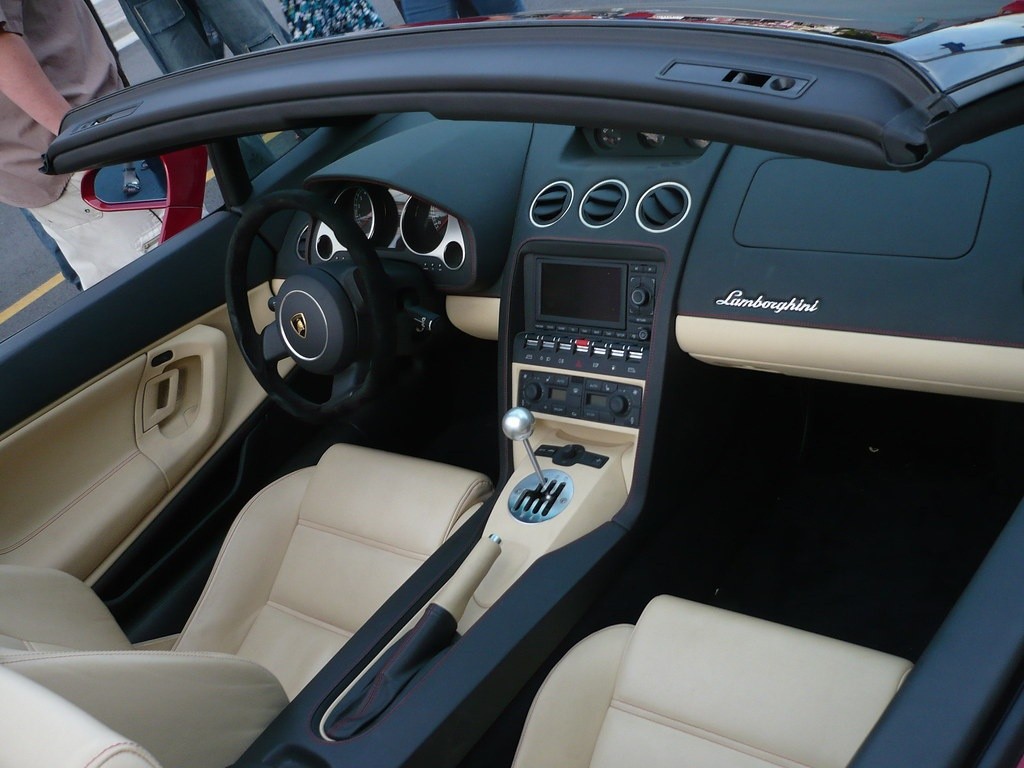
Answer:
[120,159,149,196]
[394,0,529,24]
[0,0,287,290]
[279,0,384,42]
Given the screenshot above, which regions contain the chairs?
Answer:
[512,593,918,768]
[0,439,501,768]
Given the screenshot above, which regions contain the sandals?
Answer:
[141,160,150,170]
[121,167,141,196]
